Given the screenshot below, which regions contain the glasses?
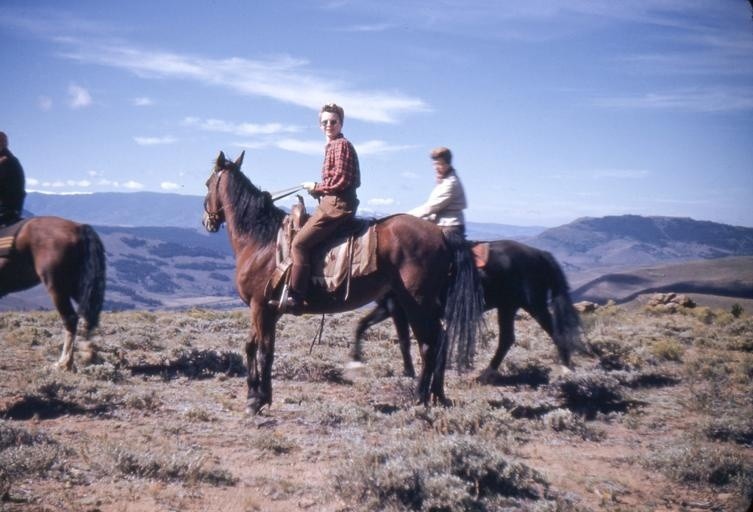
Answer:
[321,120,338,125]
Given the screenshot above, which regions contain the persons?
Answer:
[404,145,469,239]
[266,103,361,310]
[0,129,26,230]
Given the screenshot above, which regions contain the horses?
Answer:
[201,149,489,418]
[0,215,106,373]
[352,238,597,386]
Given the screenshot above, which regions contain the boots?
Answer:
[268,263,311,313]
